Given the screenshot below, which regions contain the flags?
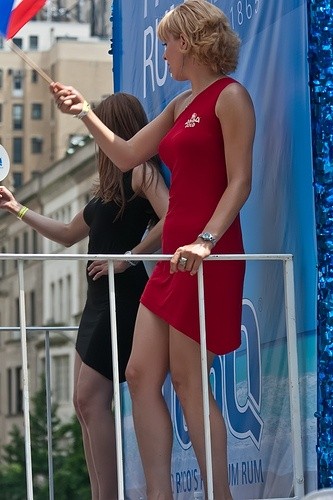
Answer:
[0,0,48,41]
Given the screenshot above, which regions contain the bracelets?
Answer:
[76,104,91,120]
[17,206,29,220]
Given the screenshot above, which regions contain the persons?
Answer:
[49,0,256,500]
[0,93,169,500]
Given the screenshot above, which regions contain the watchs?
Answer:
[123,251,149,260]
[198,232,216,246]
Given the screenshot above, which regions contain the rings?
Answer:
[179,257,187,264]
